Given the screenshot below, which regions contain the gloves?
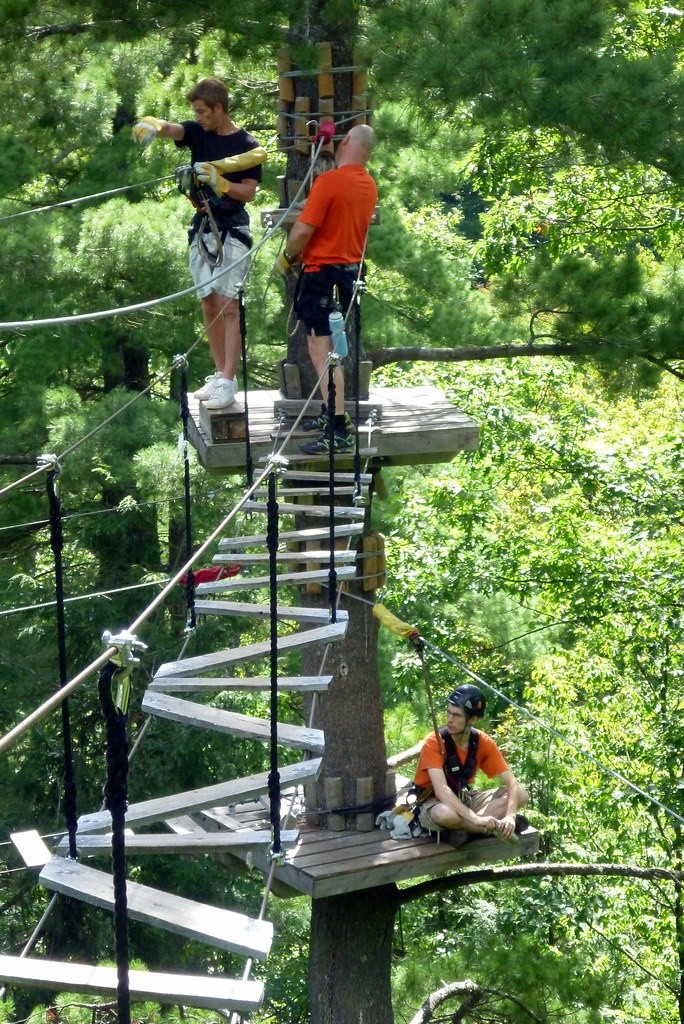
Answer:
[277,250,301,276]
[195,161,230,193]
[132,116,163,145]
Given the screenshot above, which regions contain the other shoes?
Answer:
[515,813,528,833]
[442,828,469,847]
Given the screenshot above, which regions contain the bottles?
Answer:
[329,309,348,359]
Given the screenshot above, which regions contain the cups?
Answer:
[492,827,519,847]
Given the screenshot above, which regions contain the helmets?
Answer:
[447,683,486,718]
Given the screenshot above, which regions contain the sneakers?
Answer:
[301,432,355,455]
[303,404,354,431]
[204,383,235,409]
[194,372,223,399]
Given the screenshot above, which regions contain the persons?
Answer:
[132,79,263,409]
[277,124,376,454]
[411,684,529,849]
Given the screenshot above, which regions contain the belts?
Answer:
[331,261,360,271]
